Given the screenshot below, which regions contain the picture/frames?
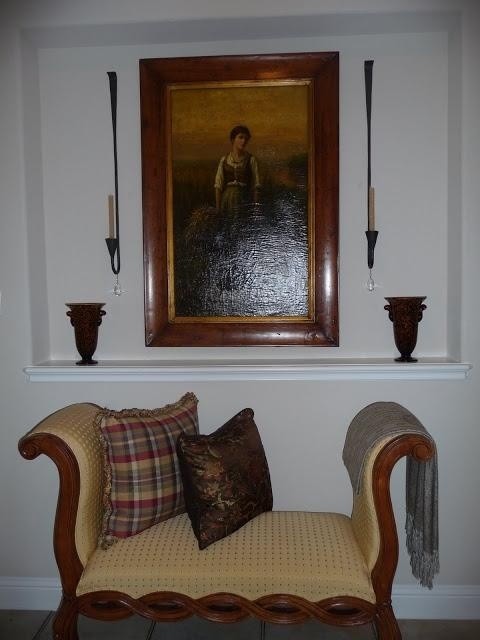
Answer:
[138,51,341,348]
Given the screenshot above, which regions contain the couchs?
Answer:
[16,400,442,636]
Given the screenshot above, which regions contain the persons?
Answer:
[213,125,263,224]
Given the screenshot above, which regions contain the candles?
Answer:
[108,193,115,238]
[369,187,375,230]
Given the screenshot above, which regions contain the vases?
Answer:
[66,301,106,366]
[384,295,429,364]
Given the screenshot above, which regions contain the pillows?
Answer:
[97,390,202,546]
[172,406,280,550]
